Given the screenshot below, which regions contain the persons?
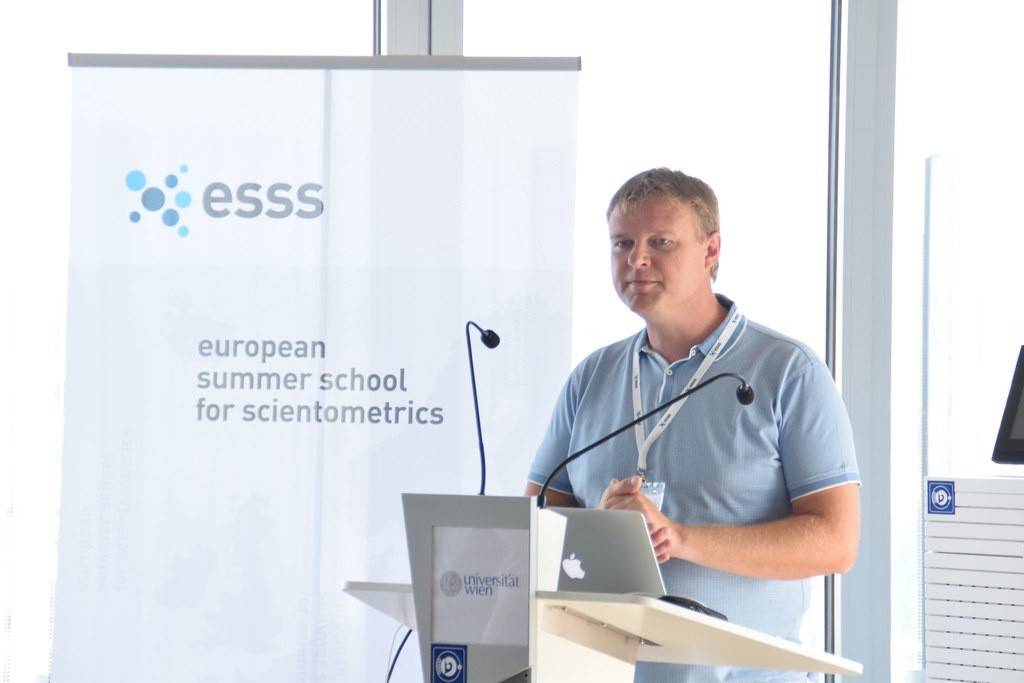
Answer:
[522,167,863,683]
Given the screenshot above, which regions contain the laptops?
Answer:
[543,505,667,600]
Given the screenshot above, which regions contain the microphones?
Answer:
[466,321,500,494]
[537,373,755,510]
